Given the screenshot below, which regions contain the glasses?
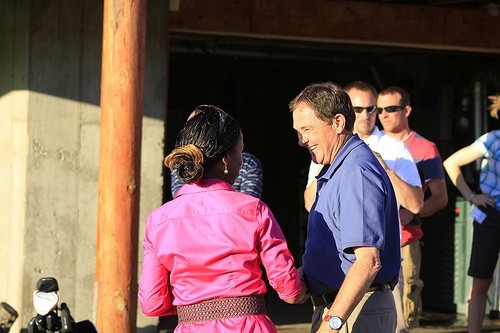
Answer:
[376,105,405,115]
[353,105,375,114]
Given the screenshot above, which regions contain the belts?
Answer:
[310,280,390,307]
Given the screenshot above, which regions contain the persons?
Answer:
[139,104,308,333]
[289,82,449,333]
[443,95,500,333]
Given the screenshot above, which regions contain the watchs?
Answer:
[324,315,344,329]
